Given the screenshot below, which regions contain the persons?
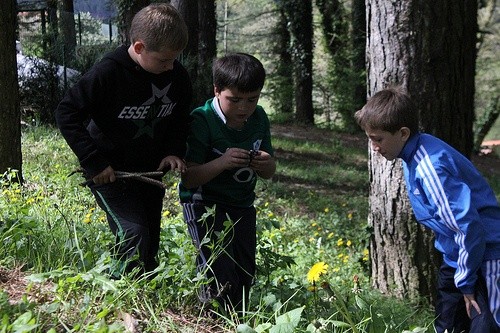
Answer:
[351,88,499,332]
[188,54,277,319]
[55,2,197,282]
